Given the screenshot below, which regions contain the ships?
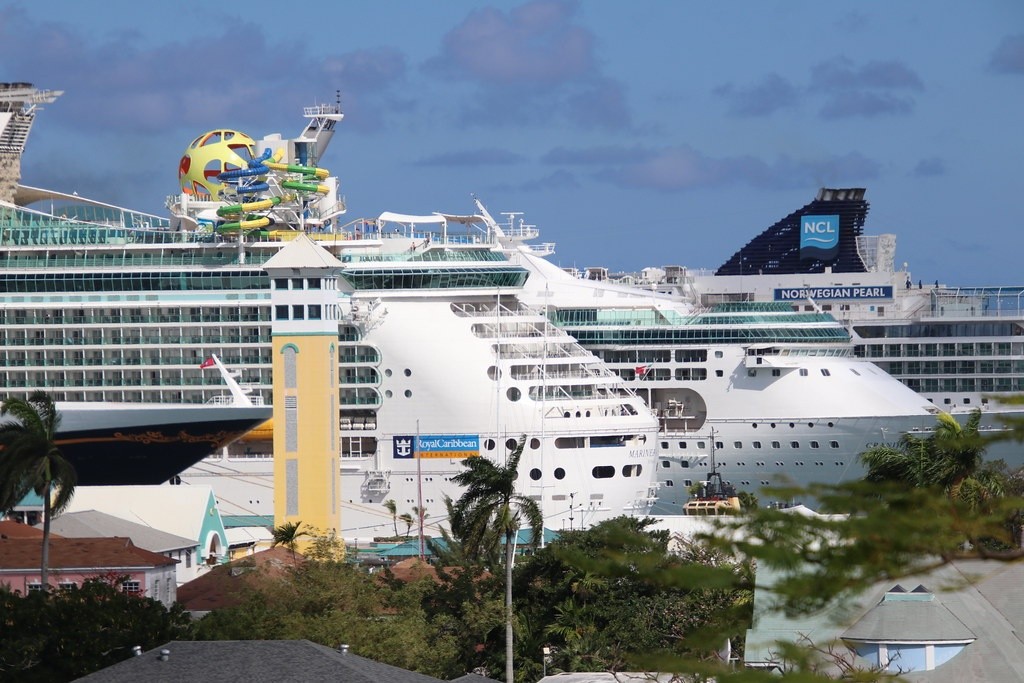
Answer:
[549,302,965,527]
[0,73,664,546]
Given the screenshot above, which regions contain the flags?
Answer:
[635,365,647,375]
[200,358,216,369]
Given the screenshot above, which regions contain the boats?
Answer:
[1,354,275,486]
